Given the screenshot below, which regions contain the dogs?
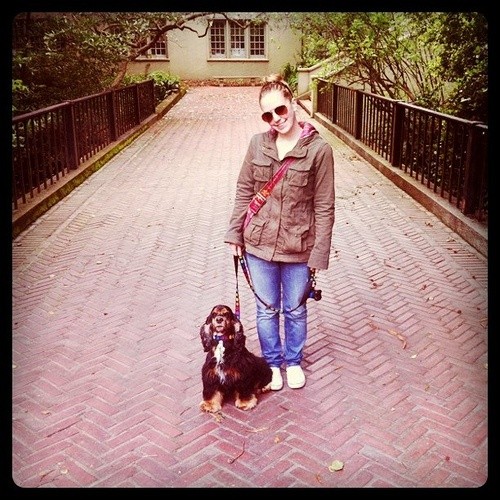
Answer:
[199,305,274,414]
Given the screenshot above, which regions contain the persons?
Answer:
[224,74,336,392]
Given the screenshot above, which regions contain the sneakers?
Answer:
[286,364,305,389]
[269,365,283,389]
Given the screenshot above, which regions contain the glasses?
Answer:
[262,101,292,122]
